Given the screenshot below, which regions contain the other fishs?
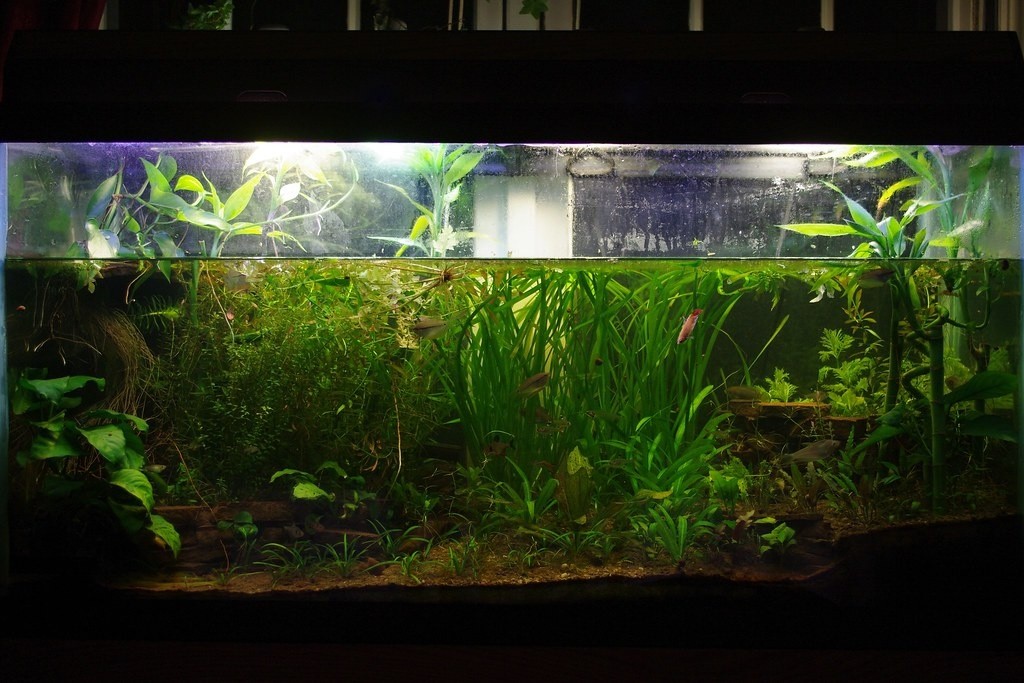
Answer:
[409,319,450,339]
[536,419,571,434]
[146,464,167,474]
[284,524,304,539]
[479,435,516,458]
[804,389,830,402]
[516,372,549,394]
[516,404,552,424]
[676,309,703,345]
[858,267,897,282]
[724,386,763,400]
[583,408,621,423]
[784,440,840,464]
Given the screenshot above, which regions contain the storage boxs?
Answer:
[1,28,1024,683]
